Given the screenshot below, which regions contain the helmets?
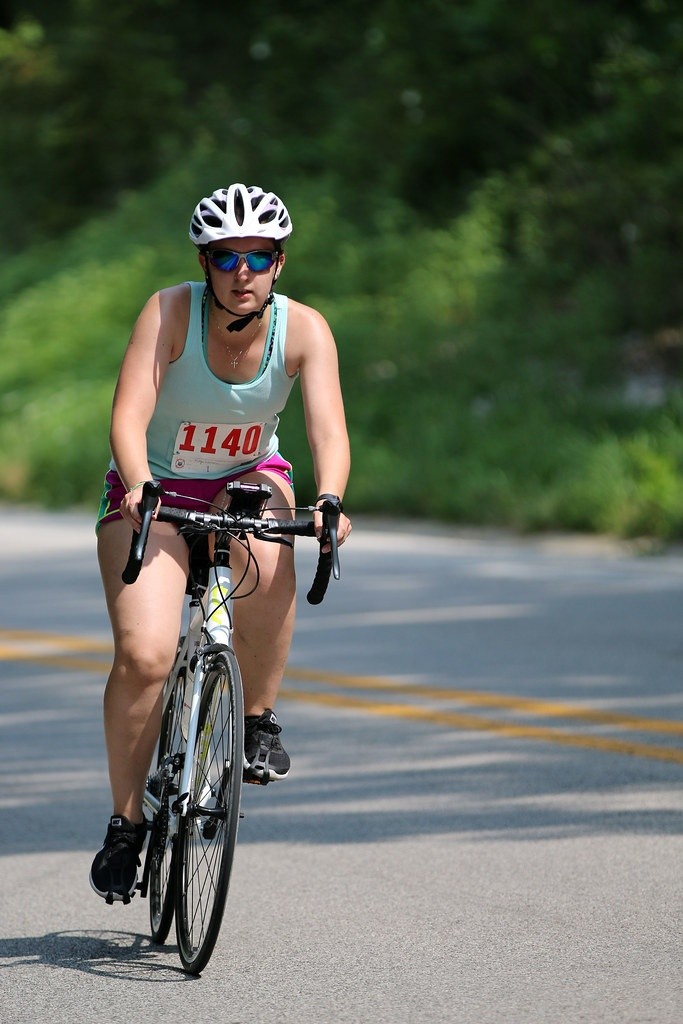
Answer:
[188,183,293,253]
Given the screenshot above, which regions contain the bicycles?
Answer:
[120,479,344,976]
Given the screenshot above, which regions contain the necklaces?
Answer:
[212,296,264,368]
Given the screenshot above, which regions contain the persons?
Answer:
[89,182,354,900]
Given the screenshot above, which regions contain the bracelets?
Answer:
[315,494,339,505]
[126,481,146,493]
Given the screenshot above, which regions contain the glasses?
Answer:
[198,248,281,272]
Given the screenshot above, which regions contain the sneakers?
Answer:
[244,708,291,781]
[89,812,147,900]
